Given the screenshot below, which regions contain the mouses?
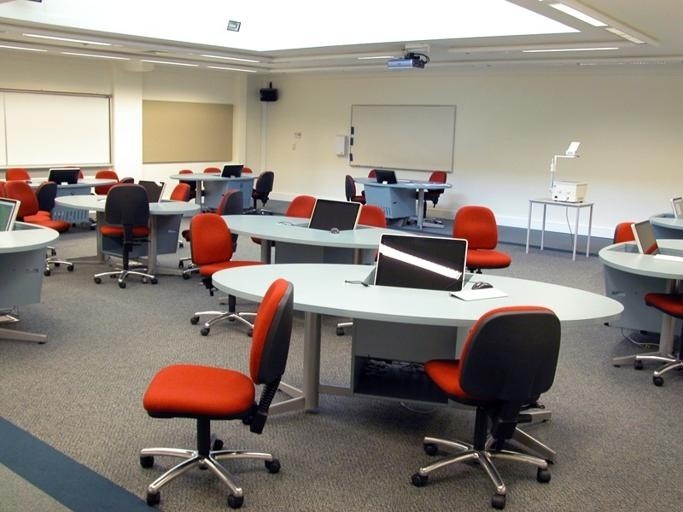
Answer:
[472,281,492,290]
[331,228,340,235]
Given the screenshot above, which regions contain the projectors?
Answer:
[384,53,431,70]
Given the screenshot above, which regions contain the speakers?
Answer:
[259,87,279,103]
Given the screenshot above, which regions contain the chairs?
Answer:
[369,170,376,179]
[285,195,317,219]
[411,305,561,510]
[453,206,512,274]
[139,279,293,509]
[415,171,446,224]
[345,175,365,204]
[358,204,387,230]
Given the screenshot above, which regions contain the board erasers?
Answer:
[350,153,352,161]
[350,138,353,145]
[351,126,354,134]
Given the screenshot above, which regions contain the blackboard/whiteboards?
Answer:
[0,88,113,171]
[349,103,456,172]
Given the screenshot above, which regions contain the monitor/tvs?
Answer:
[47,167,81,184]
[374,234,469,291]
[630,218,659,256]
[670,195,682,218]
[220,163,244,179]
[0,196,21,231]
[136,179,166,203]
[375,169,398,184]
[308,198,363,230]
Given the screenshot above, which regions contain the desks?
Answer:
[221,215,417,263]
[212,264,625,463]
[354,178,452,231]
[526,197,594,261]
[598,212,683,387]
